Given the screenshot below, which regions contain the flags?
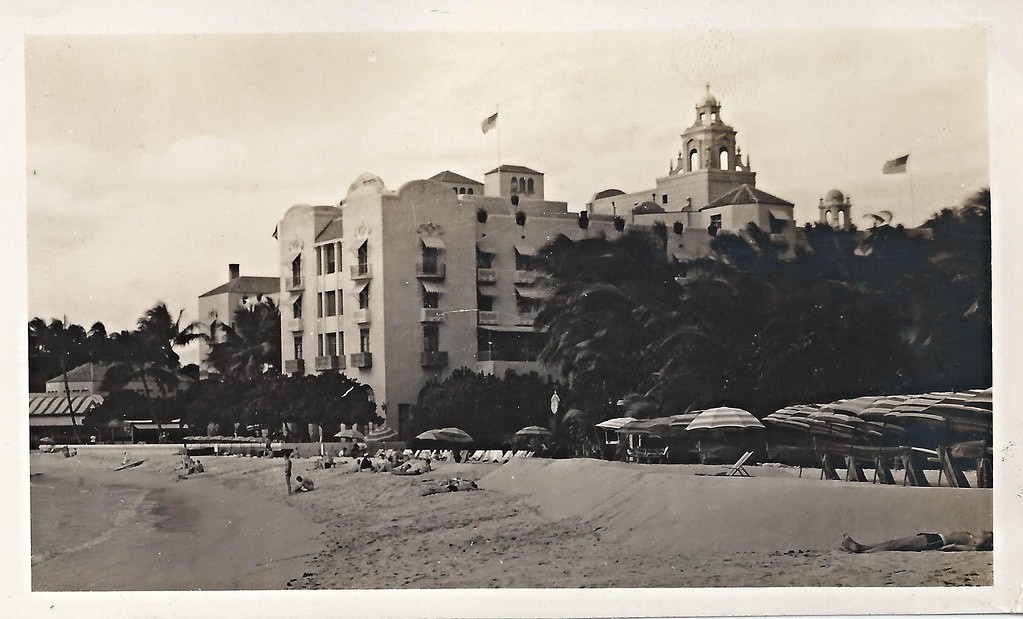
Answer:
[883,154,909,174]
[481,112,498,134]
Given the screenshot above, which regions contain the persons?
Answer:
[70,447,77,457]
[122,431,484,496]
[841,530,994,554]
[90,434,97,445]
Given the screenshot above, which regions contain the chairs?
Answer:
[722,451,754,477]
[374,449,535,464]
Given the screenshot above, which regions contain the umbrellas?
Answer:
[334,378,992,489]
[40,436,56,450]
